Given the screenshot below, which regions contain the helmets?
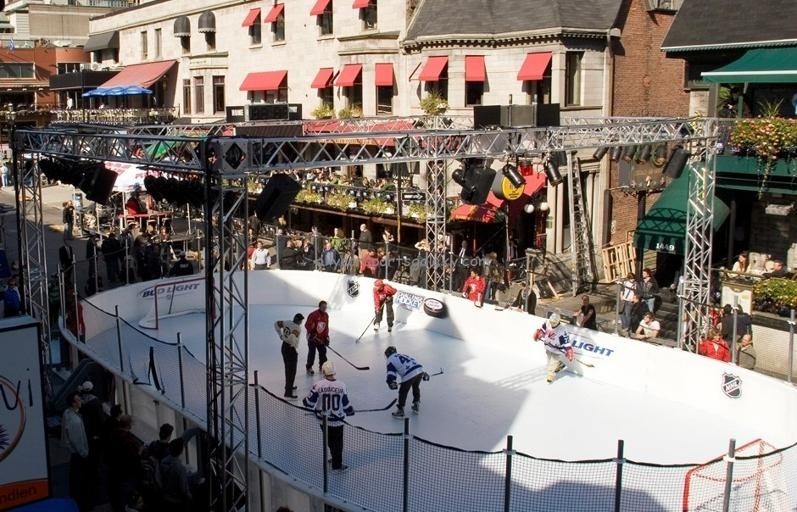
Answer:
[321,360,336,378]
[548,312,561,328]
[384,346,397,359]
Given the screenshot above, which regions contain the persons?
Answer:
[235,209,511,307]
[613,272,644,332]
[634,311,661,340]
[634,267,658,317]
[731,250,751,274]
[696,295,755,370]
[510,278,536,315]
[532,313,574,381]
[12,273,29,316]
[303,300,330,376]
[273,313,304,399]
[572,294,597,330]
[759,259,786,278]
[56,380,207,511]
[383,346,429,417]
[371,279,397,332]
[4,278,20,317]
[57,192,194,296]
[301,361,355,472]
[0,162,8,188]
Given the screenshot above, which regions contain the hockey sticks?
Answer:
[304,398,397,416]
[397,368,442,385]
[354,300,387,344]
[316,338,370,370]
[537,338,593,368]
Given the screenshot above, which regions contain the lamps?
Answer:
[136,164,254,220]
[37,158,116,205]
[590,139,669,170]
[449,152,565,195]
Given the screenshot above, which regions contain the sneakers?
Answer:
[341,464,348,470]
[374,323,379,330]
[388,327,391,332]
[308,368,314,374]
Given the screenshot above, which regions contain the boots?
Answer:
[392,407,404,416]
[411,401,420,411]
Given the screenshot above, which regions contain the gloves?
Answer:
[565,348,573,362]
[534,329,544,343]
[389,382,398,391]
[423,372,430,382]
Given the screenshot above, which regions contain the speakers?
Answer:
[85,166,119,207]
[461,166,496,205]
[254,173,304,225]
[661,146,692,180]
